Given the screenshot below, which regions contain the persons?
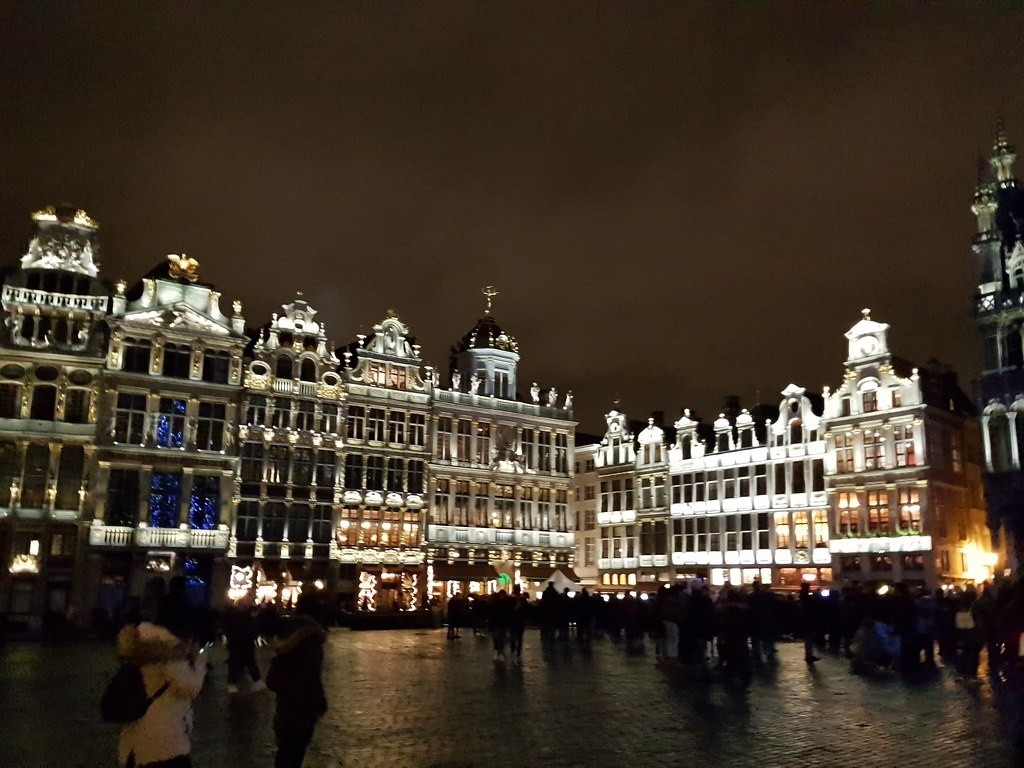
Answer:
[447,569,1024,679]
[222,611,267,694]
[116,605,208,767]
[273,593,329,768]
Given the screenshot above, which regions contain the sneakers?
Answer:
[228,679,267,694]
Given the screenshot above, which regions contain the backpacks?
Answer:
[101,663,167,721]
